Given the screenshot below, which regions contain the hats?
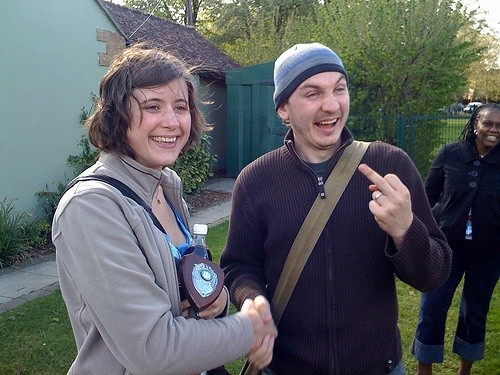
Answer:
[272,42,349,111]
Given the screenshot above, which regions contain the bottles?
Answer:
[188,224,209,264]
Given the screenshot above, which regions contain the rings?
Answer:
[373,192,382,202]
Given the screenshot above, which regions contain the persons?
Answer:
[412,103,500,375]
[220,40,456,375]
[51,50,277,375]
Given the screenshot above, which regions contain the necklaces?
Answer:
[150,185,161,204]
[479,151,485,158]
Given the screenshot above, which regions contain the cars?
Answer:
[437,102,483,115]
